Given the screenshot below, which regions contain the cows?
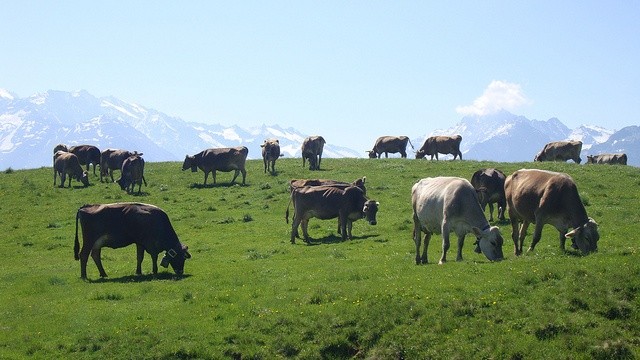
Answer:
[365,136,415,159]
[533,141,582,166]
[70,145,101,175]
[74,201,191,280]
[260,137,280,173]
[301,135,326,172]
[116,155,147,194]
[411,176,504,265]
[100,149,143,183]
[52,150,89,189]
[504,168,600,256]
[415,135,463,161]
[585,154,627,165]
[471,168,507,221]
[286,186,379,244]
[182,146,248,185]
[286,177,367,230]
